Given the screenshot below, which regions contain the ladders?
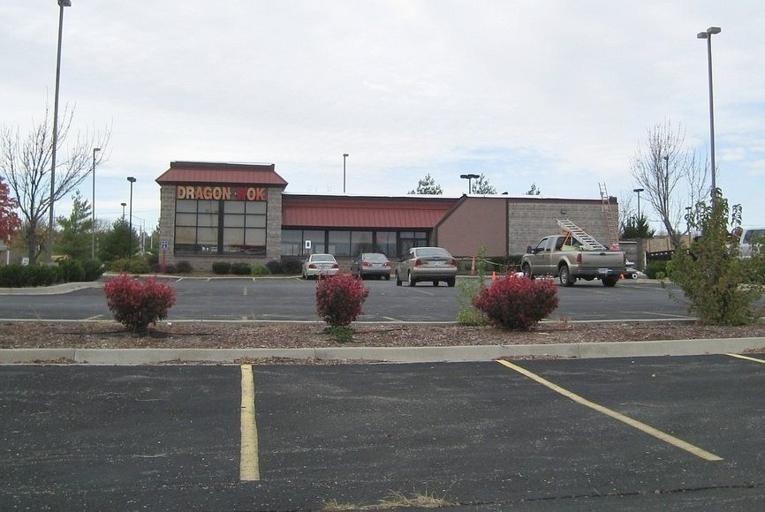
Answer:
[599,182,620,251]
[555,218,607,251]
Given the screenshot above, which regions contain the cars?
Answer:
[302,253,341,280]
[351,252,394,281]
[394,247,458,287]
[622,266,646,279]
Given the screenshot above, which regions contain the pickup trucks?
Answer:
[520,233,626,286]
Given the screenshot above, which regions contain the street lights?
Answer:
[46,0,72,266]
[461,174,480,193]
[343,154,349,194]
[91,147,100,262]
[121,203,127,224]
[697,27,721,217]
[633,188,645,227]
[127,176,136,260]
